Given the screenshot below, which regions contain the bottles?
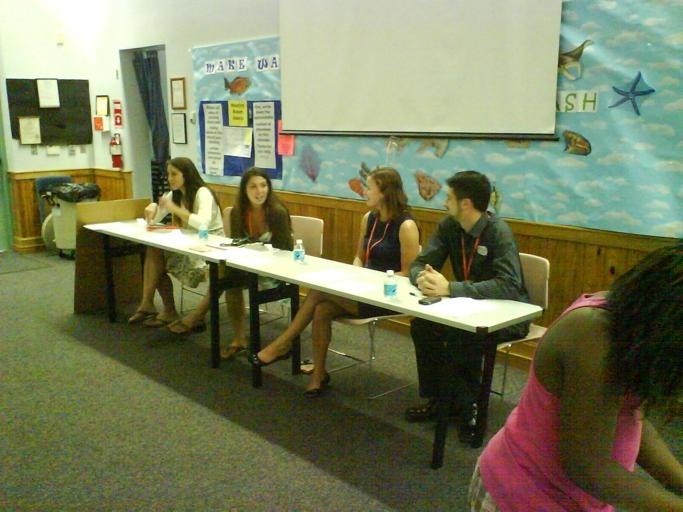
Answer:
[384,270,397,297]
[199,220,208,241]
[291,239,307,263]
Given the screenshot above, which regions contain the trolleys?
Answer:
[40,186,101,264]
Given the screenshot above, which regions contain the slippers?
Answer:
[142,312,183,328]
[164,317,208,337]
[124,309,159,324]
[216,341,249,360]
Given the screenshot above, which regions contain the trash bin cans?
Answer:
[41,182,98,260]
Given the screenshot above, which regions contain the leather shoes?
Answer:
[301,369,331,398]
[247,346,294,366]
[406,395,452,421]
[456,399,483,446]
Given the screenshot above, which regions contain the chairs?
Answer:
[326,243,421,401]
[488,253,551,403]
[180,208,232,314]
[245,215,324,328]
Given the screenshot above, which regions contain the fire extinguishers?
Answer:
[110,133,123,168]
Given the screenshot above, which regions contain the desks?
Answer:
[84,218,542,470]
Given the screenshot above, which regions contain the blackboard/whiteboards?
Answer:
[279,2,564,141]
[8,79,93,146]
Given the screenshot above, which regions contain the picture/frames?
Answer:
[96,95,109,116]
[170,77,186,109]
[171,113,187,144]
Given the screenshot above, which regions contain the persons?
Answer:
[124,157,226,331]
[163,166,295,361]
[248,168,420,400]
[465,239,683,512]
[405,170,534,446]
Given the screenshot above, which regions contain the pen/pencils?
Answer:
[410,292,424,299]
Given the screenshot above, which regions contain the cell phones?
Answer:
[232,236,245,246]
[419,294,442,305]
